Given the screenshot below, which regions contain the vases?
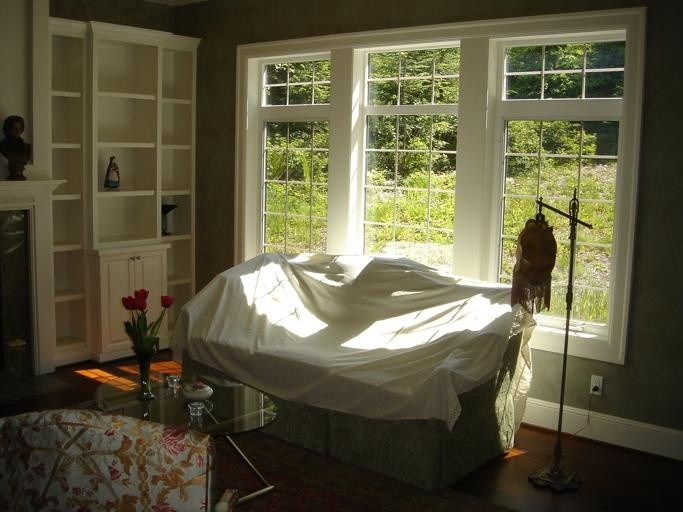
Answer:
[132,347,155,401]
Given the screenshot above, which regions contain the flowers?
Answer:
[120,286,173,386]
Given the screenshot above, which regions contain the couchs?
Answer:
[183,254,535,492]
[0,410,242,511]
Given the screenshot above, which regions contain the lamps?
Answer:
[509,186,586,492]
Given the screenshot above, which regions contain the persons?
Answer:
[0,114,33,181]
[104,153,121,191]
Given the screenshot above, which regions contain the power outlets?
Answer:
[589,374,605,398]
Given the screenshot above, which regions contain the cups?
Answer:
[187,418,203,429]
[188,401,205,416]
[165,375,178,388]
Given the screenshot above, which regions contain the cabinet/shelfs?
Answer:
[159,33,198,338]
[88,23,161,245]
[48,16,94,368]
[97,245,166,358]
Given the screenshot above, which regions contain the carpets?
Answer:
[51,379,518,512]
